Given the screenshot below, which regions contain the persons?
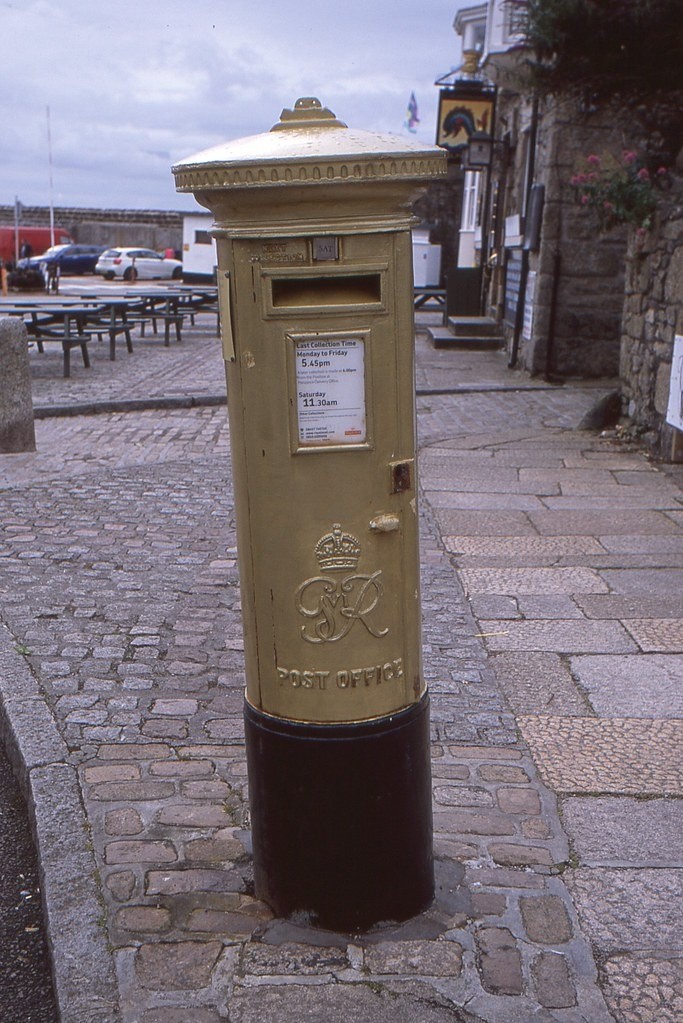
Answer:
[18,237,34,259]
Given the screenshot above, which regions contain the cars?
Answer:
[15,244,109,276]
[95,247,183,281]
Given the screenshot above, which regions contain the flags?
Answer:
[403,90,423,135]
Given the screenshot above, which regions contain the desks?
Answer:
[414,287,447,307]
[0,286,219,376]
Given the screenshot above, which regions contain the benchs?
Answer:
[52,329,109,334]
[414,304,447,312]
[127,314,189,318]
[44,324,134,328]
[104,318,150,323]
[163,310,198,315]
[26,336,91,342]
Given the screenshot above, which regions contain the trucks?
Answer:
[0,226,78,267]
